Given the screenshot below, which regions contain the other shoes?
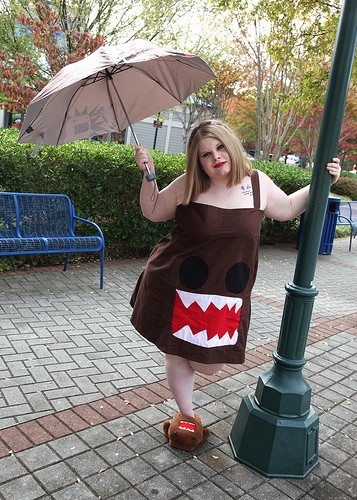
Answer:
[163,413,209,452]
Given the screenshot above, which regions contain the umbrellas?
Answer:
[16,37,220,202]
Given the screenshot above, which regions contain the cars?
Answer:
[250,150,273,162]
[278,154,301,167]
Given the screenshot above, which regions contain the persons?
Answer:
[128,118,342,453]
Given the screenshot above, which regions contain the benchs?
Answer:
[0,192,105,289]
[336,201,357,251]
